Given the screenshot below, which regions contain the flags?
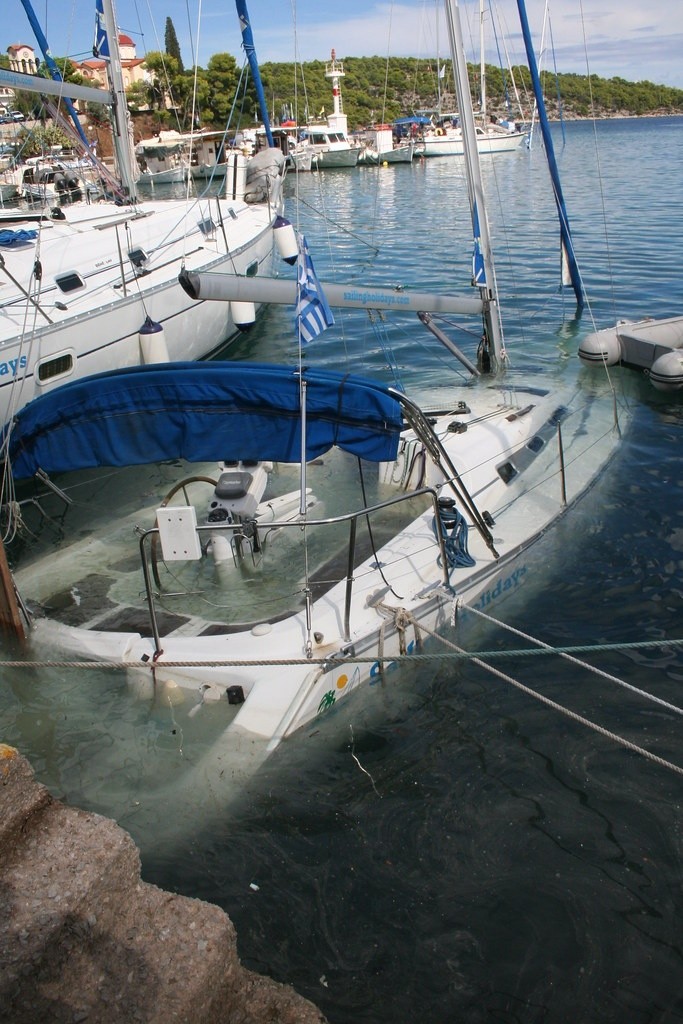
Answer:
[294,237,334,342]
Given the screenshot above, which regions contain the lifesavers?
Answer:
[434,128,443,136]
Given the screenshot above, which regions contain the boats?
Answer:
[1,0,645,866]
[0,1,287,486]
[402,0,533,157]
[134,107,415,187]
[13,143,108,196]
